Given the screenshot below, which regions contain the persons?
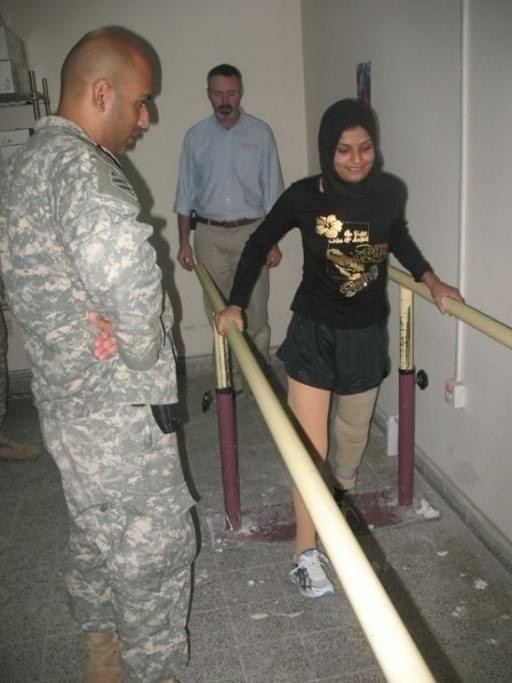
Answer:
[0,25,202,681]
[211,97,465,599]
[172,62,287,407]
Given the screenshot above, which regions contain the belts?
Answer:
[197,217,254,228]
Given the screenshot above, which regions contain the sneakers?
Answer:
[289,548,335,598]
[0,433,42,464]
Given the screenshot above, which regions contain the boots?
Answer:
[84,630,124,682]
